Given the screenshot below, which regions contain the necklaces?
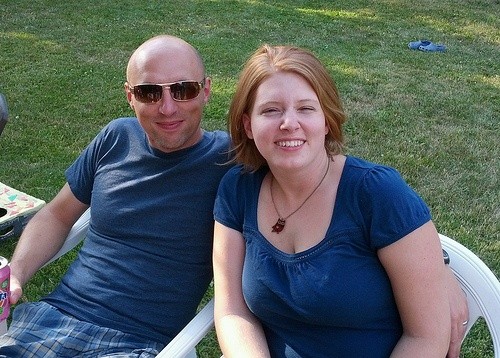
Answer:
[272,156,334,235]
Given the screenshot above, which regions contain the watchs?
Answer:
[441,249,450,265]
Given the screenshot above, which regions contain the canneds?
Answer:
[0,256,10,322]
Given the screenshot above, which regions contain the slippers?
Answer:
[408,40,446,52]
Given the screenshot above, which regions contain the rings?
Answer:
[462,320,468,326]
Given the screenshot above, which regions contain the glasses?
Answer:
[128,78,205,104]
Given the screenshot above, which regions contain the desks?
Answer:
[0,181,46,241]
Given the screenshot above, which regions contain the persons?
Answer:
[0,35,468,357]
[211,43,450,358]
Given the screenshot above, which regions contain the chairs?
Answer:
[8,206,500,358]
